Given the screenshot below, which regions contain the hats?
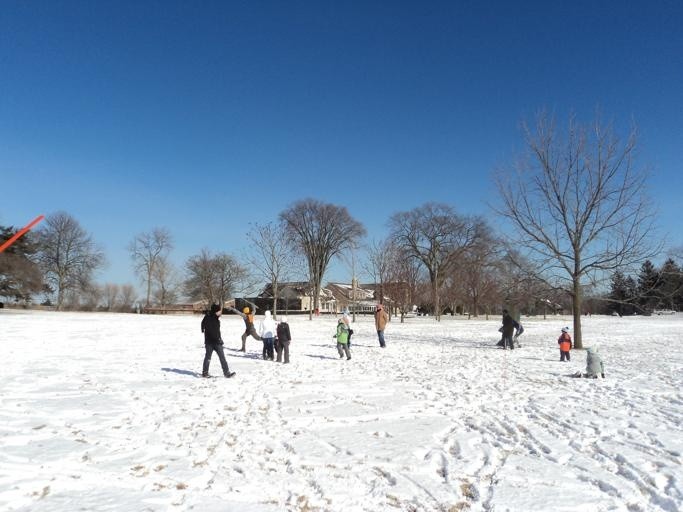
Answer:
[338,317,349,324]
[561,327,569,332]
[281,316,287,322]
[587,344,596,353]
[211,304,221,313]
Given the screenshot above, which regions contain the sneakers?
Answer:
[226,372,236,378]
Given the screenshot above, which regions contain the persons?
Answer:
[201,303,236,378]
[557,327,572,362]
[512,320,525,349]
[342,305,355,346]
[585,345,604,379]
[276,316,293,364]
[374,302,390,348]
[499,310,513,348]
[333,318,352,361]
[226,303,263,352]
[260,309,276,361]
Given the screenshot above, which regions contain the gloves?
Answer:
[602,374,605,378]
